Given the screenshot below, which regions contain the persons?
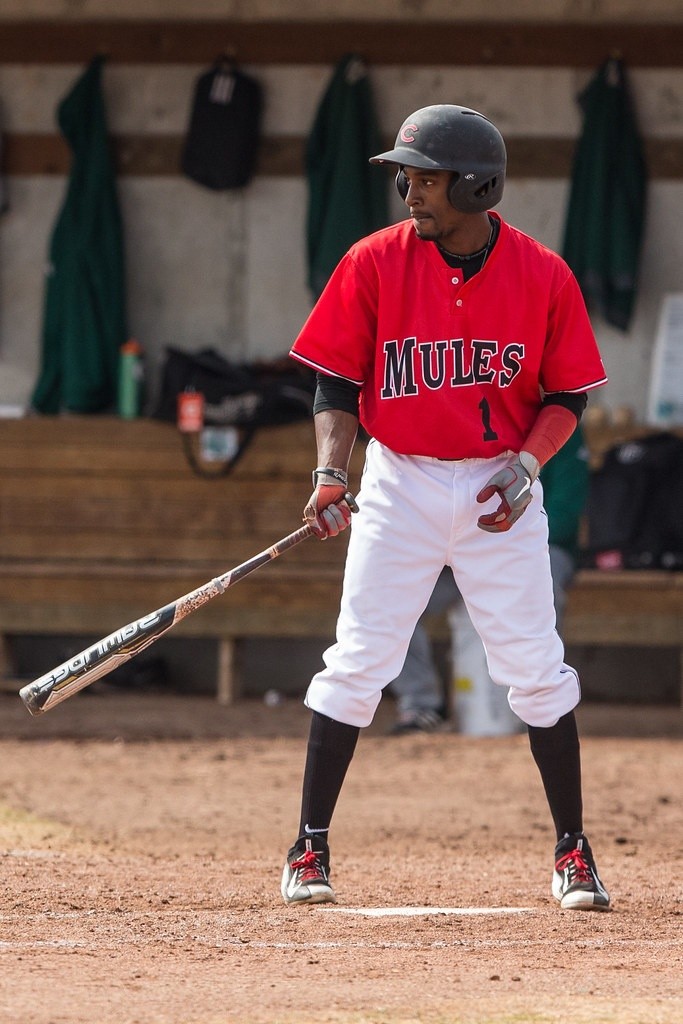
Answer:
[392,416,591,733]
[280,107,616,913]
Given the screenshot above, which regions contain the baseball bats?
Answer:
[16,488,360,718]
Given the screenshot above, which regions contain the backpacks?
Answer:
[585,433,681,574]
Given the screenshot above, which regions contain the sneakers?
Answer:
[551,834,610,910]
[281,833,335,906]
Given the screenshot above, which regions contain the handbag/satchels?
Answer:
[152,344,313,475]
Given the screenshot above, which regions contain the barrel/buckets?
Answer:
[448,604,523,740]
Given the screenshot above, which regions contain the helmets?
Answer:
[371,104,507,214]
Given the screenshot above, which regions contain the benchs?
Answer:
[0,416,683,703]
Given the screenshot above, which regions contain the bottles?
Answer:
[118,340,143,421]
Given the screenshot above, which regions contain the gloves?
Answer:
[304,468,352,540]
[476,454,533,534]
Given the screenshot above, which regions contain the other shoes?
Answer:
[386,709,451,740]
